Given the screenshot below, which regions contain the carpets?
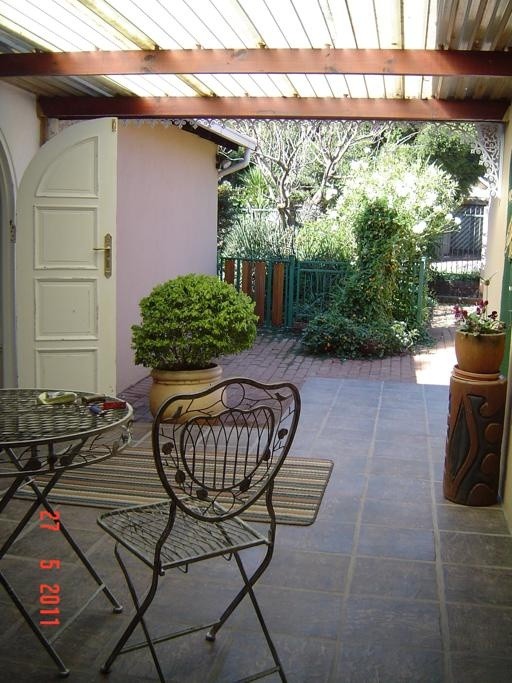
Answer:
[0,438,335,527]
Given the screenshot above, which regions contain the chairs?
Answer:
[95,376,302,683]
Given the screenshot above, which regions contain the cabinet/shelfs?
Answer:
[442,366,503,508]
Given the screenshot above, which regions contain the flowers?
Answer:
[450,272,509,340]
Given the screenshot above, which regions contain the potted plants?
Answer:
[127,268,261,420]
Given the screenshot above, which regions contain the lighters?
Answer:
[90,405,107,416]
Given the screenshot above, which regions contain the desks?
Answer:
[0,385,136,679]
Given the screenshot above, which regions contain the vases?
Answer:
[454,329,508,375]
[293,320,386,358]
